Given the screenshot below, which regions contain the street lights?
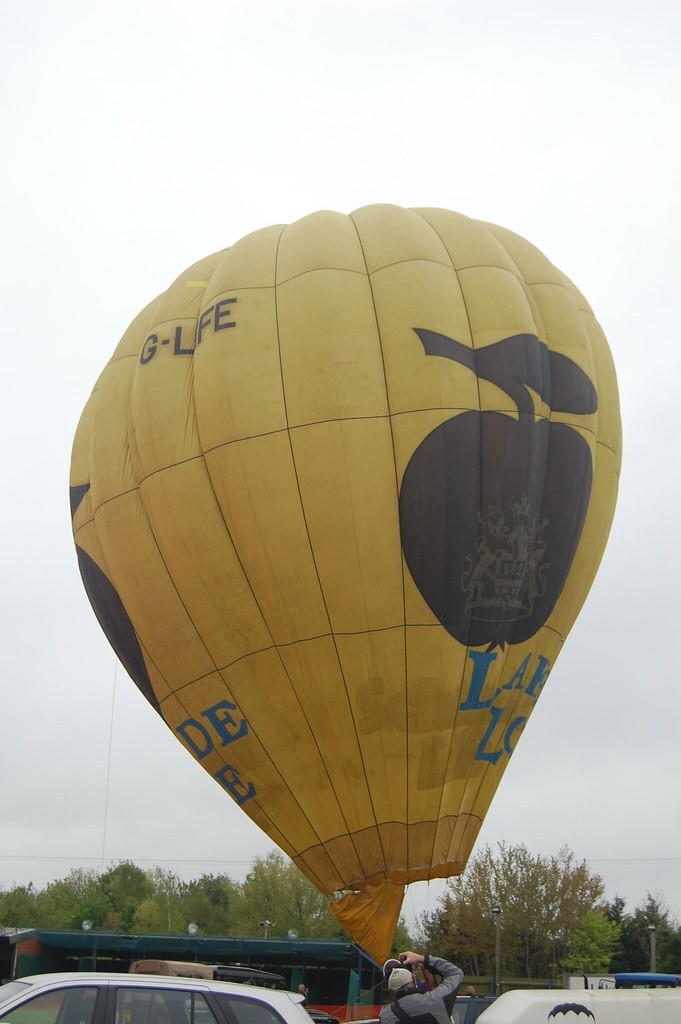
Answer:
[260,920,277,940]
[491,905,503,993]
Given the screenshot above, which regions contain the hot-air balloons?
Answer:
[69,203,623,969]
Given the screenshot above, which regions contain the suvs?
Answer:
[120,959,340,1024]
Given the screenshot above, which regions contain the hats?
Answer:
[382,959,413,991]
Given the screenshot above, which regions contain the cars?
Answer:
[0,971,314,1024]
[448,972,680,1024]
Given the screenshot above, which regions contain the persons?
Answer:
[298,984,309,1008]
[379,951,464,1024]
[465,985,476,997]
